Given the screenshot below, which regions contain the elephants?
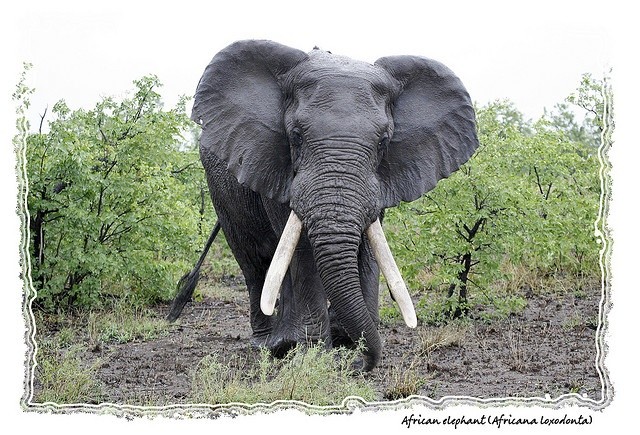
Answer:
[166,39,479,375]
